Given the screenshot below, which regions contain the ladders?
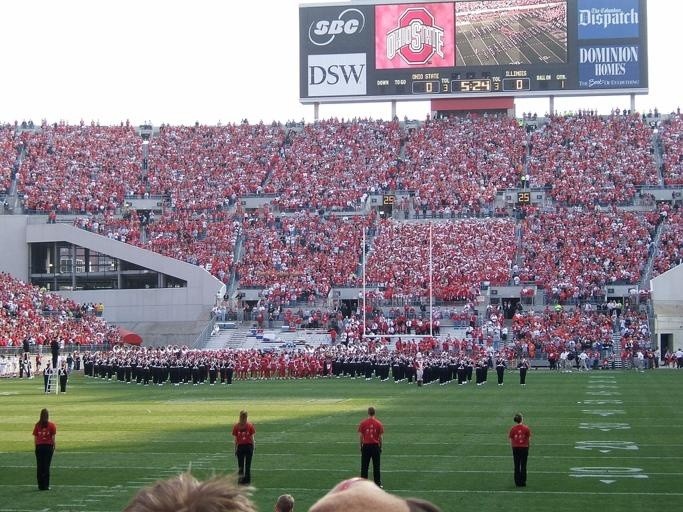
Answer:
[46,368,58,396]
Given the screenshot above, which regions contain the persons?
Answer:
[356,406,384,489]
[232,409,257,486]
[43,363,53,393]
[273,494,294,512]
[123,460,258,512]
[507,412,533,488]
[1,106,681,385]
[455,0,567,61]
[31,407,57,490]
[58,364,67,394]
[308,476,443,512]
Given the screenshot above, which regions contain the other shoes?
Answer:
[84,374,170,386]
[520,383,525,385]
[376,376,487,386]
[498,382,503,386]
[231,374,372,380]
[170,378,232,386]
